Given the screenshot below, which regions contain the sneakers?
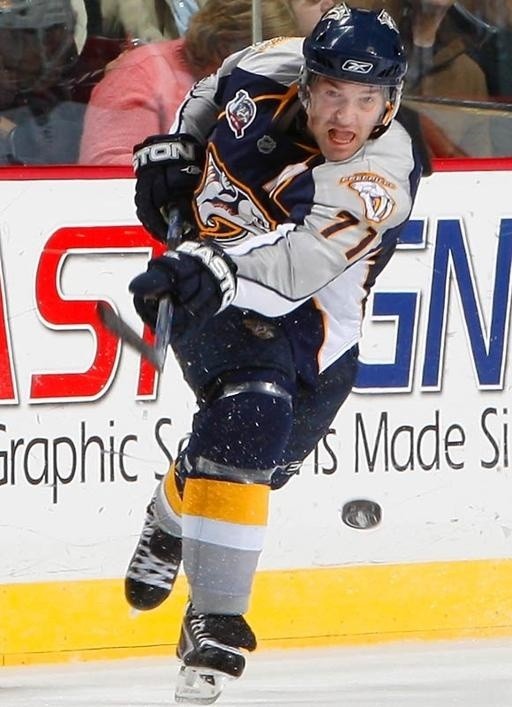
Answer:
[176,600,257,680]
[125,495,182,611]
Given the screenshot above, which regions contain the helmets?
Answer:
[298,2,409,142]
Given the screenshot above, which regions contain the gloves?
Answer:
[133,134,204,243]
[129,237,237,351]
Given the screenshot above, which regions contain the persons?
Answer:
[127,3,424,676]
[1,0,512,166]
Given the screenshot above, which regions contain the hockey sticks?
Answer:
[97,200,185,373]
